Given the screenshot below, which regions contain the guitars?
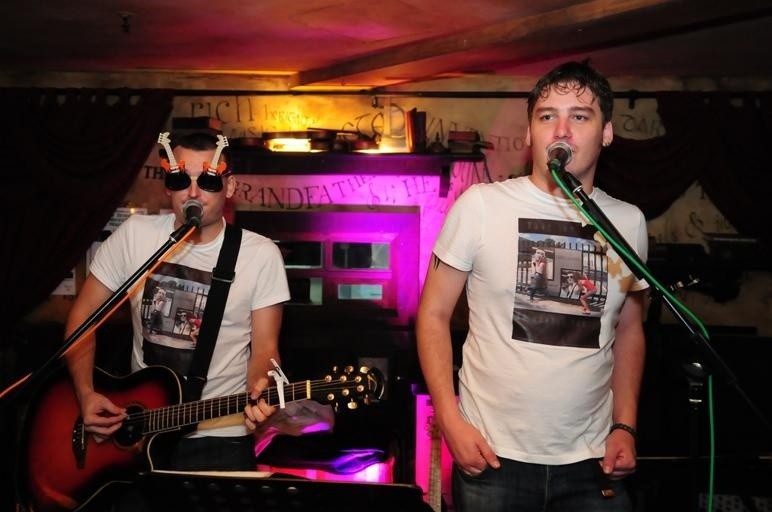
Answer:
[23,357,387,511]
[158,132,191,191]
[197,134,229,192]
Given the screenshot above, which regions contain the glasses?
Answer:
[158,131,231,193]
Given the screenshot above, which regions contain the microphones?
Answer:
[545,140,572,172]
[182,200,205,228]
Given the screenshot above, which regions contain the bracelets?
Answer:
[609,422,639,441]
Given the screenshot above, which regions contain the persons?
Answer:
[572,272,597,315]
[173,311,193,336]
[524,253,545,301]
[559,271,581,300]
[187,311,201,348]
[145,293,166,334]
[414,61,654,509]
[61,131,294,476]
